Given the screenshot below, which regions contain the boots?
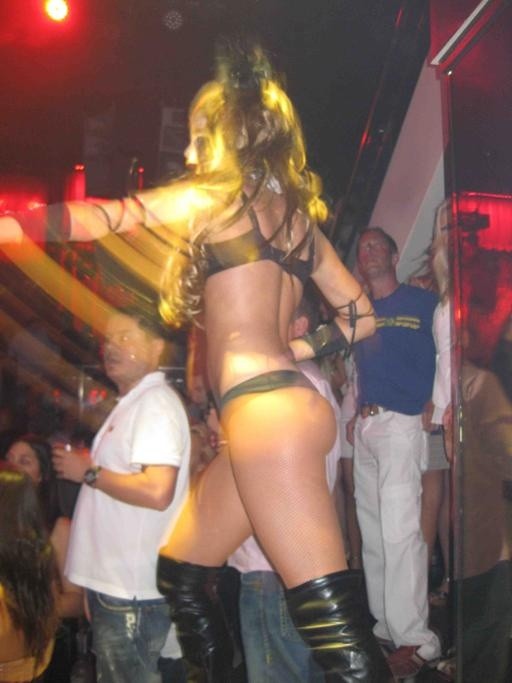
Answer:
[280,565,398,683]
[154,550,237,681]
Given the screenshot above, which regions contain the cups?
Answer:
[50,439,86,451]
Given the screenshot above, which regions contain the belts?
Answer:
[357,400,393,420]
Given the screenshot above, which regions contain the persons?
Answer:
[0,71,390,683]
[1,194,511,682]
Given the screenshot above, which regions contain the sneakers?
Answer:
[386,637,428,681]
